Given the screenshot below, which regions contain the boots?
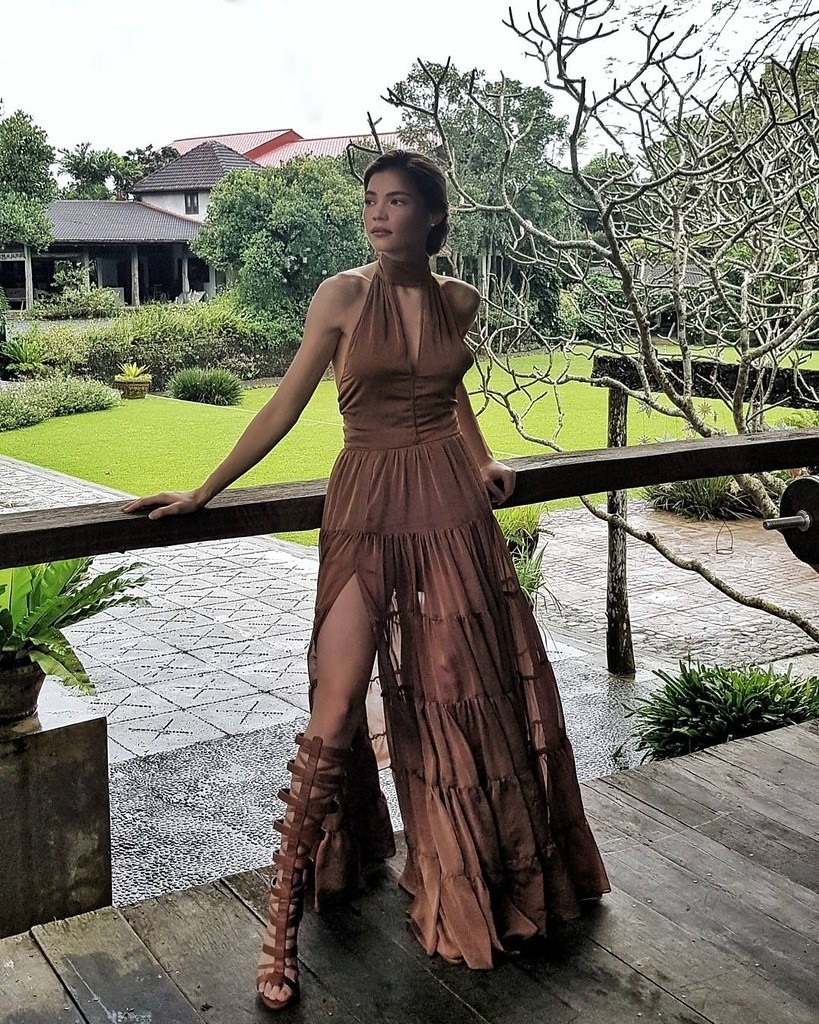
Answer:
[251,733,354,1012]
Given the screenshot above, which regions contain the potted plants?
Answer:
[493,502,549,562]
[115,361,151,400]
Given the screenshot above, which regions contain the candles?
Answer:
[1,552,155,724]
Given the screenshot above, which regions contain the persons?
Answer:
[120,151,610,1011]
